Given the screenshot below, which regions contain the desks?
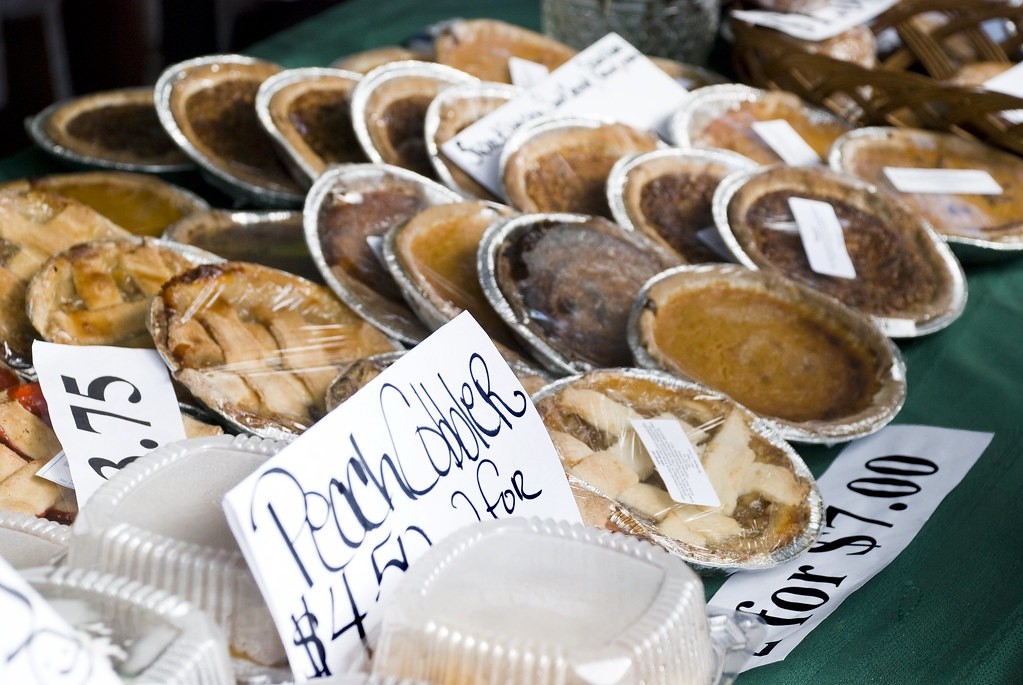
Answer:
[1,0,1021,685]
